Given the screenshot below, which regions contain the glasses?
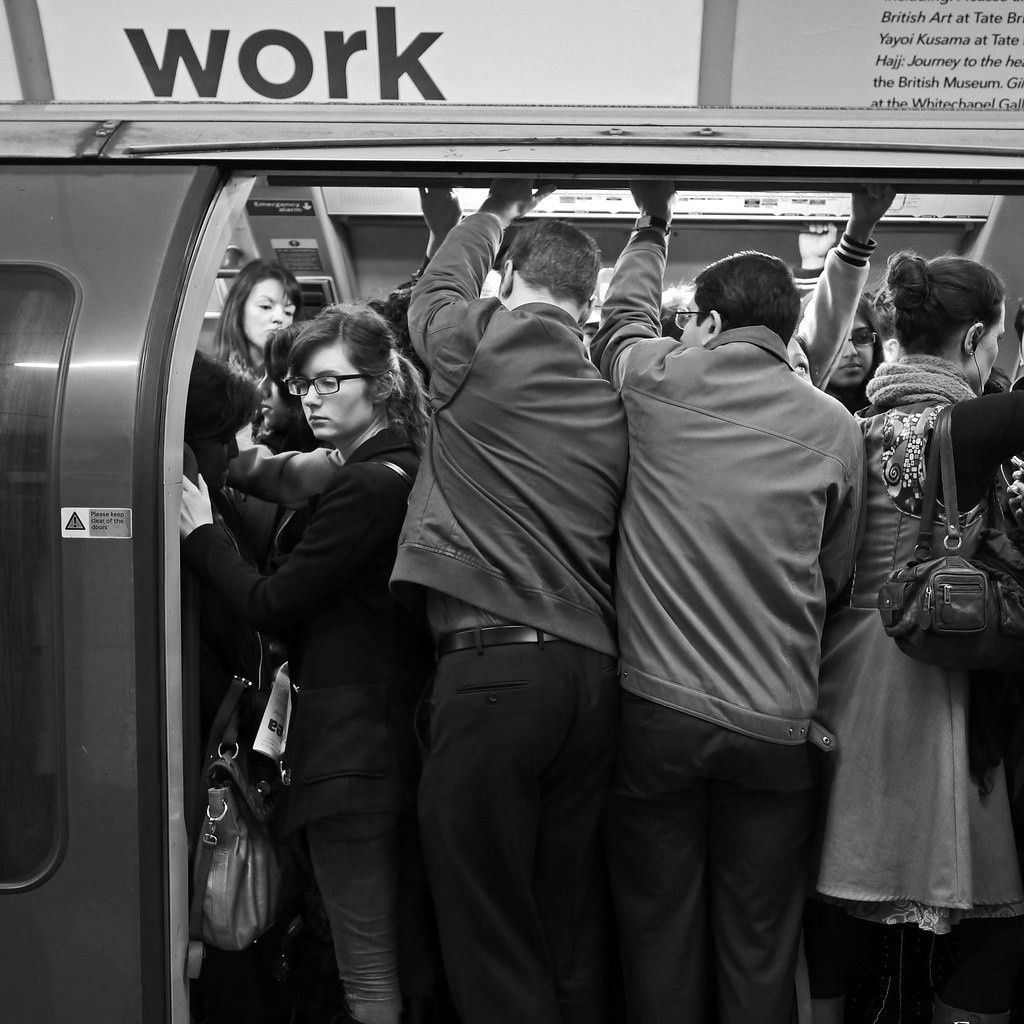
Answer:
[675,306,706,329]
[282,371,371,396]
[849,328,876,346]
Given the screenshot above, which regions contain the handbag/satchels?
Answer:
[191,751,281,952]
[879,556,996,660]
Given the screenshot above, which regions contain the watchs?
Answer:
[634,215,671,236]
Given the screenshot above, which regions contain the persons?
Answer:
[178,178,1023,1024]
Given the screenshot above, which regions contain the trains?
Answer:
[0,96,1024,1024]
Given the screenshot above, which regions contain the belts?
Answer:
[436,624,556,651]
